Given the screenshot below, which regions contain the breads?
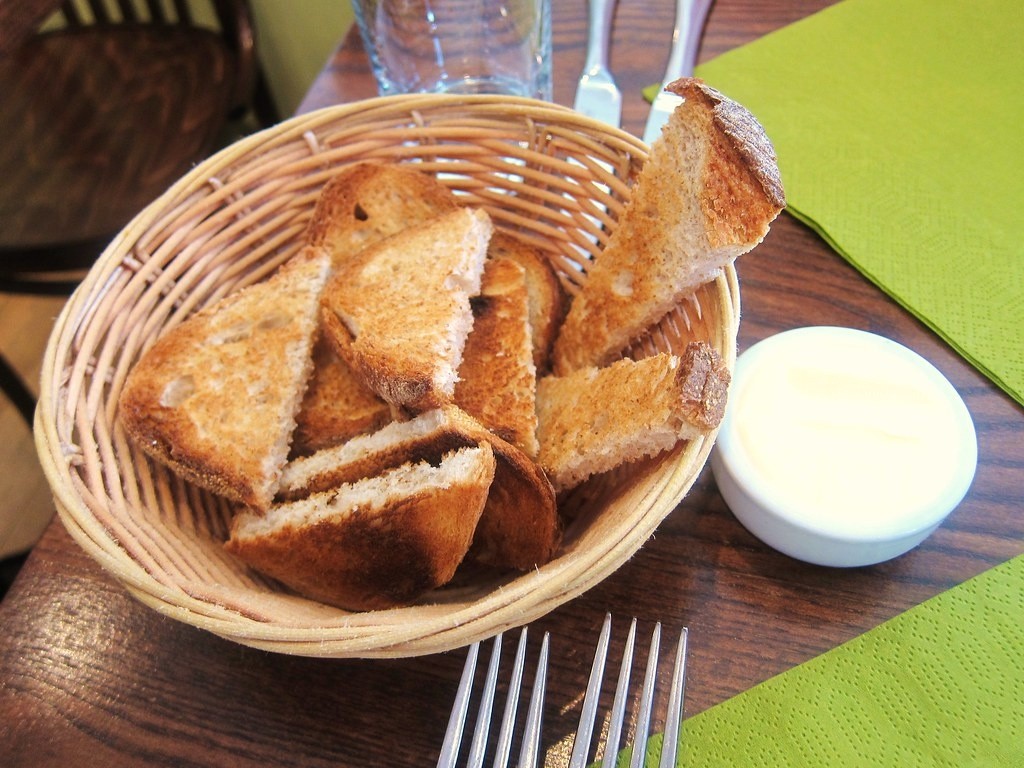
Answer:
[118,245,334,514]
[302,163,462,266]
[548,78,785,376]
[318,208,494,417]
[224,442,493,612]
[277,404,558,573]
[488,231,565,372]
[290,257,541,458]
[535,341,732,490]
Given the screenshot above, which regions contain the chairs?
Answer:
[0,0,284,430]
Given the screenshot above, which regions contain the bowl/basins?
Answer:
[712,326,979,567]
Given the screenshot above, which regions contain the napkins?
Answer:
[640,0,1023,407]
[585,552,1024,768]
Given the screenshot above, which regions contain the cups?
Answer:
[350,0,551,196]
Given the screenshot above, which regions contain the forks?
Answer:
[567,613,688,768]
[436,626,550,768]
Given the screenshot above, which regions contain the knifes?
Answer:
[557,0,622,281]
[643,0,715,154]
